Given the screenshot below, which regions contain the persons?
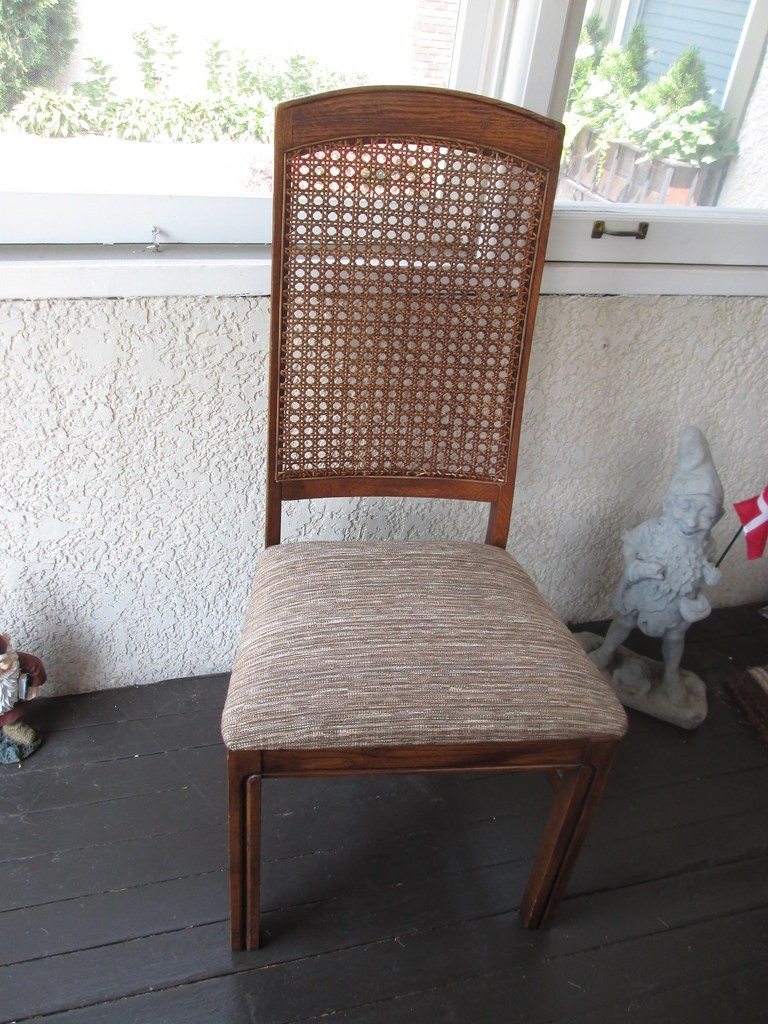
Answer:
[0,633,47,763]
[588,425,726,707]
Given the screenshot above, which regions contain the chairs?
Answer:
[218,85,630,955]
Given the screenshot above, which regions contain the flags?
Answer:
[733,485,768,560]
